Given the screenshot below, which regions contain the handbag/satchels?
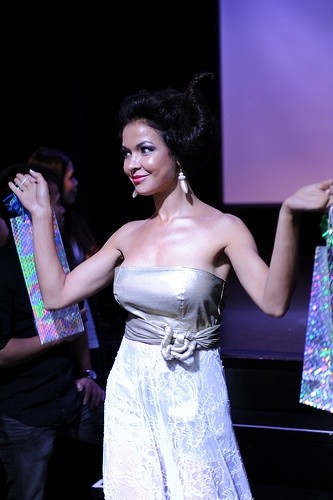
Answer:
[2,177,86,346]
[298,205,333,413]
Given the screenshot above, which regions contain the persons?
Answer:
[8,87,333,500]
[0,148,105,499]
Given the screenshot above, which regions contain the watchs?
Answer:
[81,368,97,381]
